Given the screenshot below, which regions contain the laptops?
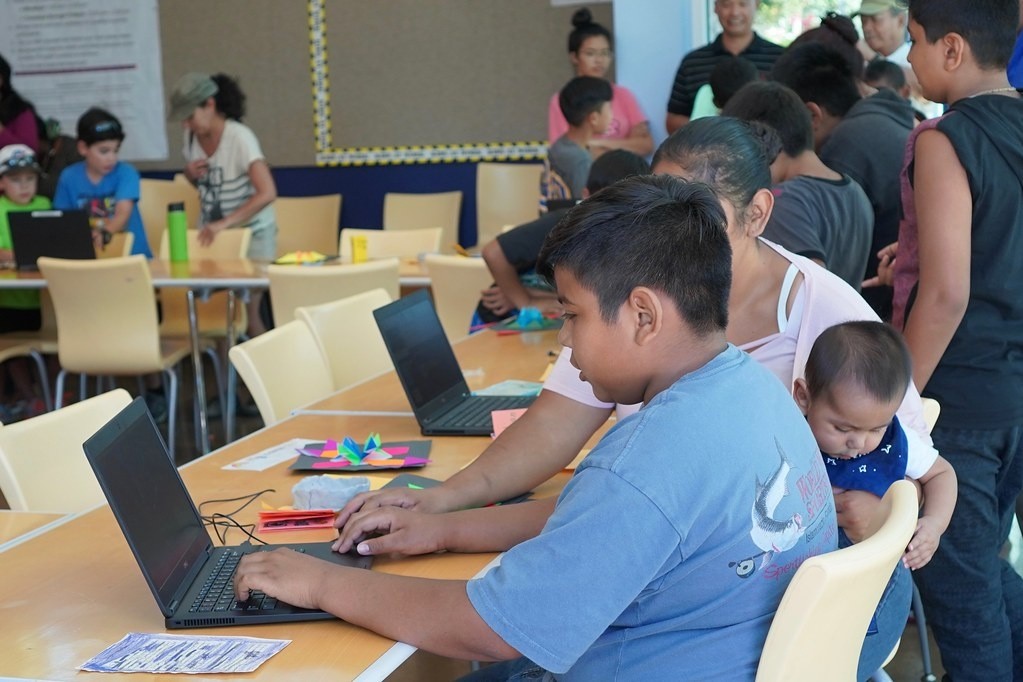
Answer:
[8,208,96,272]
[82,395,372,629]
[373,288,537,437]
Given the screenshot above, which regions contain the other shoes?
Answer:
[26,400,45,416]
[205,393,260,419]
[145,387,168,423]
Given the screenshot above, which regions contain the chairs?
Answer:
[1,163,936,682]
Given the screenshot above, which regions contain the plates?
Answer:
[270,253,340,264]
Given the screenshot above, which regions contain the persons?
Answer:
[231,172,837,682]
[481,0,1023,682]
[165,71,279,415]
[0,55,75,415]
[335,117,934,682]
[51,108,168,424]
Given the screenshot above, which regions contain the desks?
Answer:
[0,259,269,453]
[324,257,430,286]
[295,327,621,419]
[2,414,626,682]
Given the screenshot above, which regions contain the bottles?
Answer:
[166,201,188,261]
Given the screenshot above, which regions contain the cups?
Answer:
[350,235,367,263]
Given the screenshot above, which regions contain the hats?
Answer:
[0,144,47,185]
[850,0,910,19]
[168,74,219,122]
[77,108,123,146]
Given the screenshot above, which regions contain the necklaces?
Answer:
[970,87,1016,97]
